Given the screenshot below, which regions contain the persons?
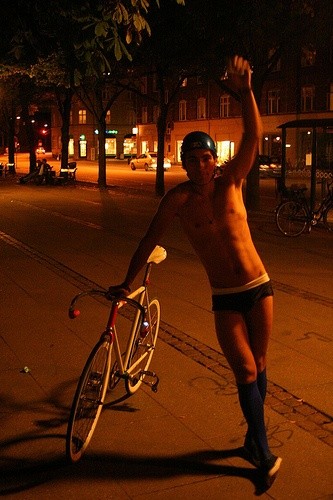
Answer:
[102,53,287,481]
[287,156,305,183]
[19,159,51,185]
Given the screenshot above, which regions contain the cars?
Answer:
[130,151,171,171]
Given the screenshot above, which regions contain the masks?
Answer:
[187,166,214,185]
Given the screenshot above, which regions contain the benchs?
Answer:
[52,167,77,188]
[0,163,15,177]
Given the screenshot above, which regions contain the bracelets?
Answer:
[120,283,132,293]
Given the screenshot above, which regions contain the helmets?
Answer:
[181,131,216,161]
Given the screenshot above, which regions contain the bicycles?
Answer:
[276,175,332,237]
[65,244,168,463]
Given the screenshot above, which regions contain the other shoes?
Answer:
[244,439,261,466]
[260,454,282,487]
